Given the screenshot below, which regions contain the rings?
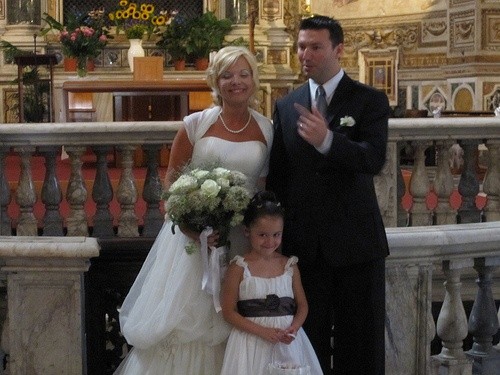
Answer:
[298,123,304,129]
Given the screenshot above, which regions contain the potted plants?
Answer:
[156,25,192,70]
[182,11,244,70]
[0,41,52,124]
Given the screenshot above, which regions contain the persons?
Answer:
[222,190,324,375]
[113,46,274,375]
[266,15,390,375]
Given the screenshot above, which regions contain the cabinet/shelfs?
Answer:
[13,53,59,124]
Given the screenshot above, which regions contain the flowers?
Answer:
[58,25,107,58]
[161,167,257,255]
[109,0,165,38]
[339,115,356,127]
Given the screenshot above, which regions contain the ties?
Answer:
[315,86,328,116]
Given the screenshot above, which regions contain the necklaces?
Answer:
[217,114,254,134]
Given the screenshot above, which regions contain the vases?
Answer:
[127,39,144,73]
[64,56,97,77]
